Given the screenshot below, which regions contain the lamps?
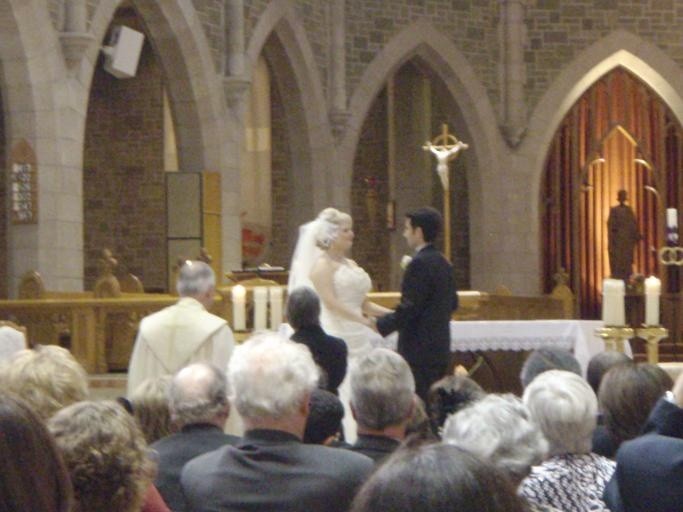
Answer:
[365,188,397,231]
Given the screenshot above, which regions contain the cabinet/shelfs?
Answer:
[163,170,221,291]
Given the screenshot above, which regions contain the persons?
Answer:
[607,189,643,280]
[287,206,395,442]
[127,257,235,397]
[0,284,683,511]
[370,207,461,399]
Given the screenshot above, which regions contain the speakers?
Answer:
[105,26,145,78]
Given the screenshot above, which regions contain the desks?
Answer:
[371,283,570,319]
[0,292,180,375]
[382,320,635,391]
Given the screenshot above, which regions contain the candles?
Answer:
[231,284,247,331]
[666,208,679,243]
[270,285,283,333]
[643,276,663,327]
[252,286,268,330]
[600,278,626,326]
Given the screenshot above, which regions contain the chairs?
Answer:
[92,275,123,298]
[18,269,48,301]
[552,284,574,320]
[495,284,512,296]
[122,272,146,294]
